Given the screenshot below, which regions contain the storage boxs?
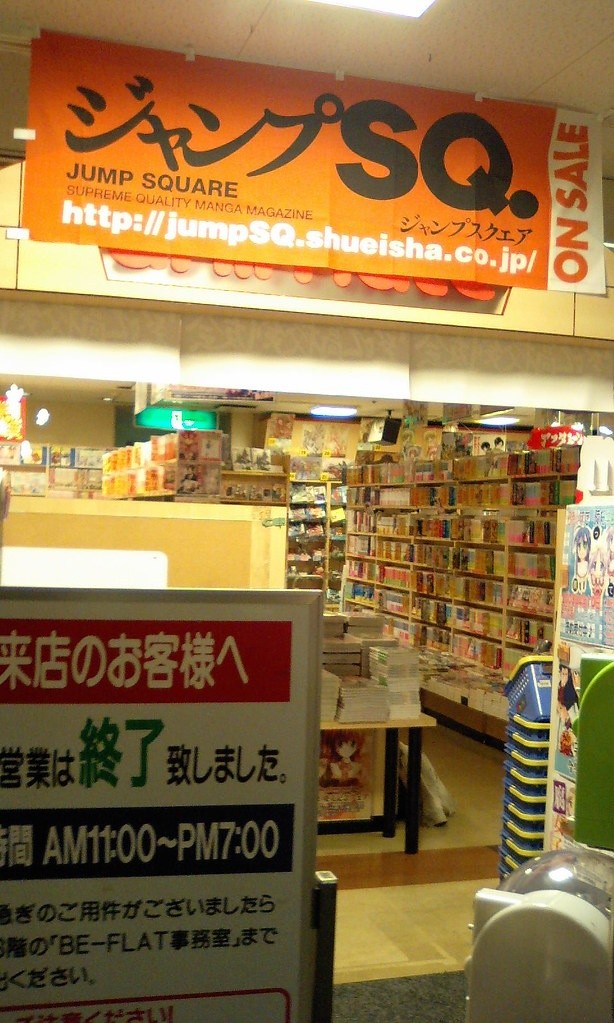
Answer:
[318,611,398,685]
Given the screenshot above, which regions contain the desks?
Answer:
[320,711,437,855]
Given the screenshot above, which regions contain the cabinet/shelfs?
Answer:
[339,446,581,677]
[0,440,128,500]
[287,453,354,611]
[102,430,288,507]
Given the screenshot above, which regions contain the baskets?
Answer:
[498,656,553,886]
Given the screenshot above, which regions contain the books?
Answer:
[339,446,580,722]
[100,427,221,502]
[547,779,578,851]
[1,442,103,496]
[555,659,588,782]
[231,411,296,472]
[552,502,614,648]
[321,608,425,721]
